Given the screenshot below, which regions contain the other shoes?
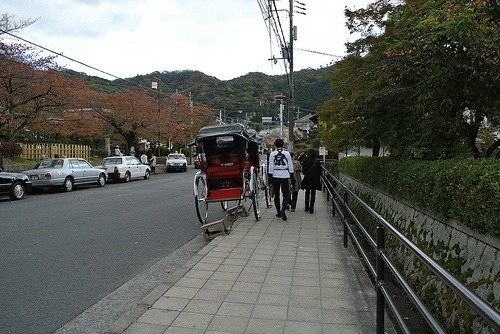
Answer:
[291,205,295,210]
[281,210,287,220]
[306,207,309,212]
[310,208,313,213]
[277,212,281,218]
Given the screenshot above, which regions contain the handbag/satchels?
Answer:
[300,181,305,189]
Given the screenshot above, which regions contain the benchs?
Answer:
[202,153,246,181]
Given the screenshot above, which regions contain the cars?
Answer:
[194,153,207,169]
[99,156,151,183]
[21,158,108,192]
[166,154,188,172]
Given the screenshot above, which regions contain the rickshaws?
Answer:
[191,122,260,237]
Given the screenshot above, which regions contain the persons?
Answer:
[286,149,322,213]
[41,155,45,161]
[268,139,296,222]
[111,145,156,175]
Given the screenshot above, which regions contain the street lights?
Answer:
[224,110,243,125]
[274,94,287,140]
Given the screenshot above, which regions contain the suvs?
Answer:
[0,167,32,200]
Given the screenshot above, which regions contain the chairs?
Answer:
[200,204,249,240]
